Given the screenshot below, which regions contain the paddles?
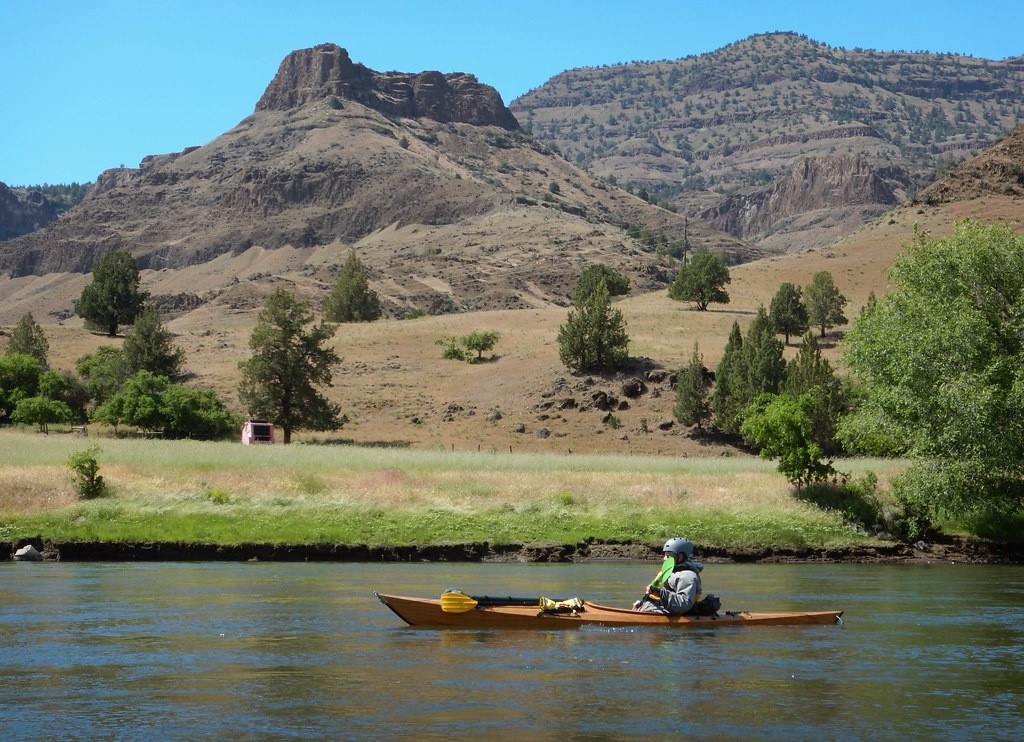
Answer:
[440,592,539,614]
[632,557,675,611]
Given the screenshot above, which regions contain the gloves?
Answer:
[648,585,660,596]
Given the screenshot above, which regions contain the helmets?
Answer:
[662,538,693,556]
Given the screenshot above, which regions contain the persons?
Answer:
[632,537,702,614]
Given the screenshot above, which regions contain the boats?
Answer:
[373,590,844,630]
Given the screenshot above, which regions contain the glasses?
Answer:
[664,553,679,558]
